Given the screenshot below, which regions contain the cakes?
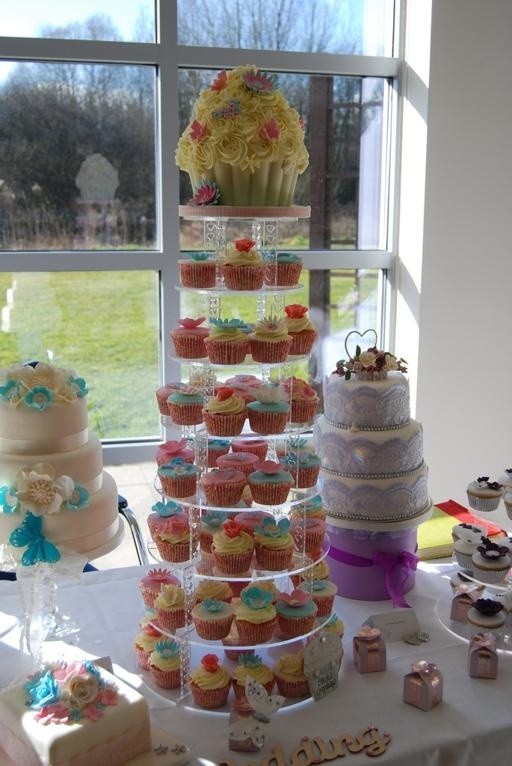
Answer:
[296,580,337,617]
[280,453,321,488]
[313,349,435,532]
[223,239,263,290]
[503,493,512,522]
[180,253,216,288]
[248,315,293,363]
[471,536,511,584]
[0,361,126,568]
[450,569,474,595]
[311,614,344,640]
[237,320,252,353]
[262,248,304,286]
[247,460,294,505]
[232,653,275,700]
[216,452,260,484]
[153,516,190,563]
[283,304,317,355]
[467,476,502,511]
[147,638,189,688]
[232,439,268,463]
[499,537,512,557]
[176,64,310,208]
[234,512,274,534]
[226,375,263,418]
[234,588,276,643]
[467,599,506,639]
[292,558,330,585]
[453,540,480,569]
[157,383,185,416]
[289,518,324,553]
[193,437,231,467]
[240,581,281,604]
[191,599,234,640]
[276,436,315,464]
[167,386,204,425]
[169,317,209,359]
[273,654,309,697]
[200,469,247,506]
[283,376,319,423]
[189,654,231,709]
[0,659,153,766]
[197,580,233,606]
[245,386,290,433]
[135,625,172,670]
[156,439,195,467]
[158,457,201,498]
[202,388,246,436]
[203,318,249,365]
[276,590,318,635]
[452,523,487,541]
[153,582,197,629]
[288,495,326,522]
[138,569,181,608]
[254,517,294,571]
[498,468,512,488]
[147,501,188,542]
[211,521,254,574]
[200,511,227,553]
